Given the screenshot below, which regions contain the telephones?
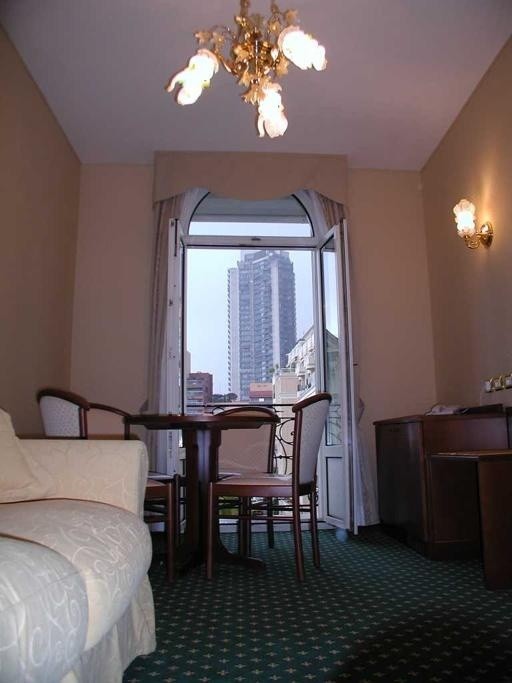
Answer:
[432,404,459,415]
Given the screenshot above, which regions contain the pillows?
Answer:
[0,409,59,503]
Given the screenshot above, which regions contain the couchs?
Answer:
[0,440,156,683]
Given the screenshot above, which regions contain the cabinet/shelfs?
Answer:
[373,414,510,560]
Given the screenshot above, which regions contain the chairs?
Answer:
[204,391,332,583]
[33,389,180,584]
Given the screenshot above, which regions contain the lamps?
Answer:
[453,199,493,252]
[166,0,328,140]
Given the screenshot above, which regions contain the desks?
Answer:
[121,411,281,585]
[428,450,512,591]
